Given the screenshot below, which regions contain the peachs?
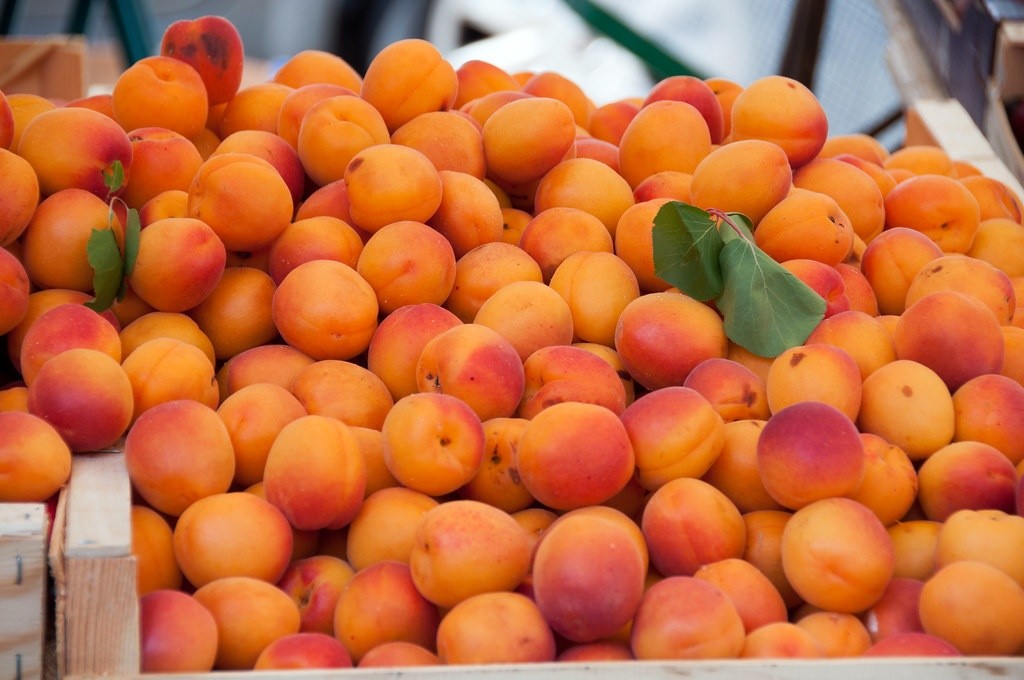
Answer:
[0,13,1024,673]
